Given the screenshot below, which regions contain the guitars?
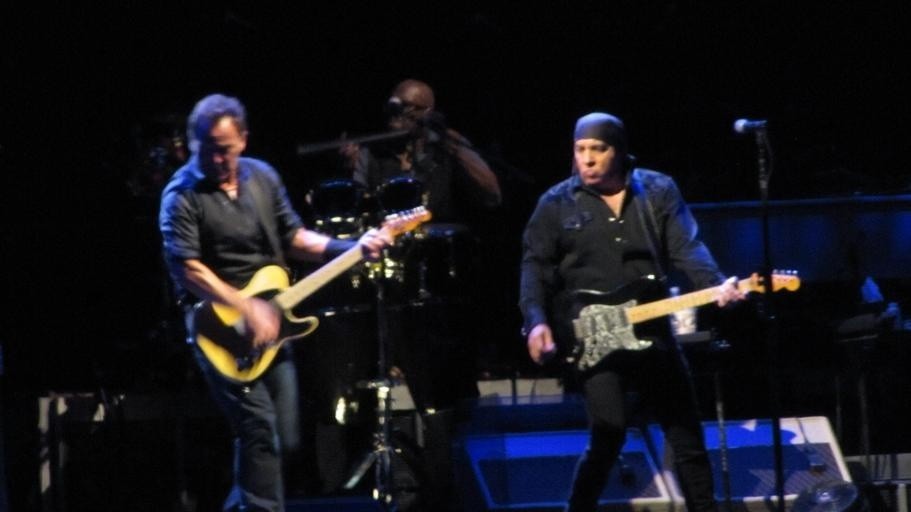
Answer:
[185,206,433,385]
[536,270,801,381]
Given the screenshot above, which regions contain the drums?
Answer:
[324,237,430,315]
[311,182,368,237]
[372,177,434,238]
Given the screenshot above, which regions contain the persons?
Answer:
[332,79,502,222]
[158,93,396,510]
[519,112,747,511]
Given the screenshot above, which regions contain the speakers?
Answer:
[473,427,673,511]
[652,415,858,512]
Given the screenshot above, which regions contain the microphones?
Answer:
[389,95,401,121]
[737,118,769,133]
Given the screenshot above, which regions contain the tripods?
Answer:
[324,217,451,512]
